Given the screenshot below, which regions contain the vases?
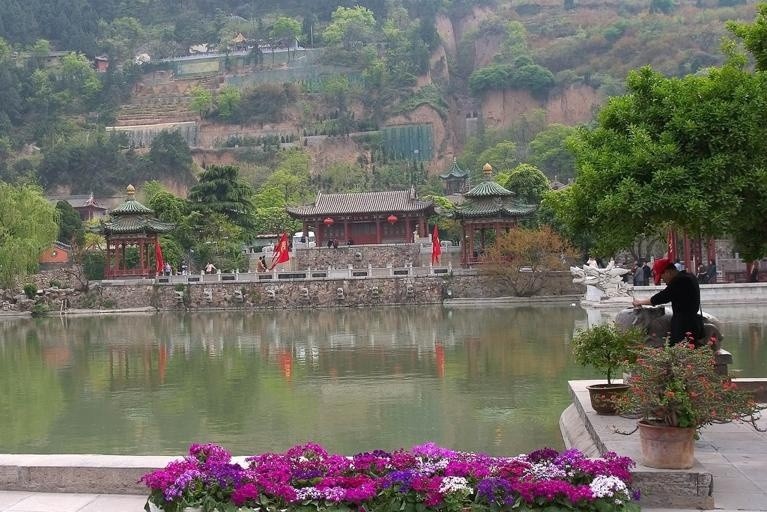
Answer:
[635,418,697,471]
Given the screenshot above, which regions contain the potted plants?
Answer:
[574,319,645,415]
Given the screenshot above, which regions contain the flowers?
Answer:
[625,336,754,428]
[135,443,642,511]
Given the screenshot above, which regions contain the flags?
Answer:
[433,338,447,379]
[269,232,289,270]
[431,222,441,266]
[276,348,292,379]
[156,343,166,383]
[154,239,165,274]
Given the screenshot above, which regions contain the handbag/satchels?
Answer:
[681,316,706,342]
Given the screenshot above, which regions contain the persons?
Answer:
[629,257,702,351]
[256,256,265,273]
[164,262,172,276]
[618,256,716,286]
[203,260,217,275]
[261,255,267,272]
[178,258,186,275]
[326,239,333,248]
[746,260,761,282]
[346,236,353,245]
[332,239,339,248]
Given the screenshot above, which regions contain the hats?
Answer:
[654,258,673,285]
